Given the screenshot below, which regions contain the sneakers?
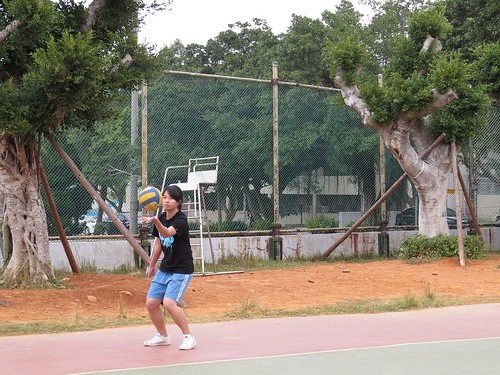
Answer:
[179,333,196,350]
[144,333,170,346]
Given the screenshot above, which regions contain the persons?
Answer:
[143,185,197,349]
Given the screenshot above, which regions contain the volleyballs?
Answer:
[138,186,163,212]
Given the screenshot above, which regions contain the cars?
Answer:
[84,215,129,237]
